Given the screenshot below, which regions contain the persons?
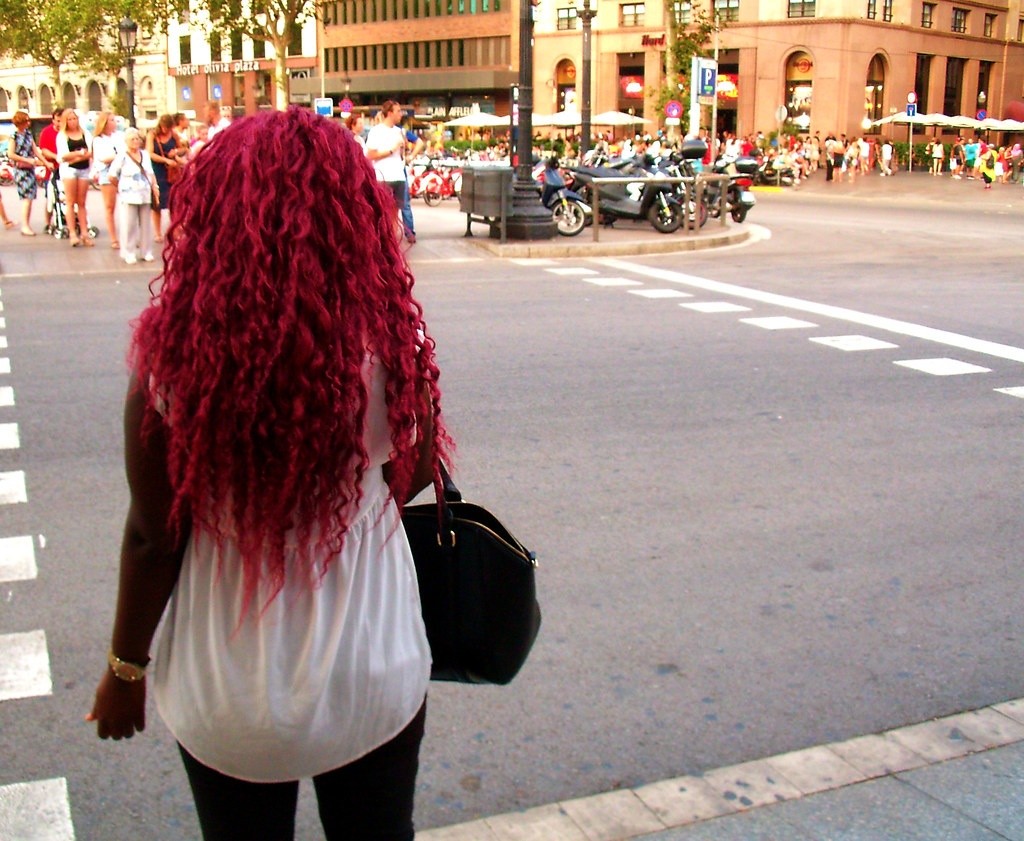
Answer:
[345,114,367,157]
[362,99,426,243]
[0,100,232,266]
[696,126,1023,190]
[87,104,458,841]
[400,129,685,171]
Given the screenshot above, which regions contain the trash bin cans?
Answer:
[458,164,514,217]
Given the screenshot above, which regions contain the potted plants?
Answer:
[895,141,951,172]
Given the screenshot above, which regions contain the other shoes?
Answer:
[407,235,416,243]
[139,250,153,262]
[20,228,35,237]
[70,234,79,246]
[4,219,16,230]
[111,239,119,250]
[80,235,94,246]
[121,253,136,265]
[155,234,164,243]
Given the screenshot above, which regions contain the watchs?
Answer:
[107,647,148,682]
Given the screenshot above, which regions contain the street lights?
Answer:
[574,0,600,162]
[118,14,138,130]
[977,91,987,139]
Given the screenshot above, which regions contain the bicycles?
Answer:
[422,163,462,208]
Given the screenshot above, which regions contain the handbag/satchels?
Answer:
[151,187,160,211]
[167,161,185,185]
[395,455,542,687]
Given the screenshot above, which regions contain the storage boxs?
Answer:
[735,157,758,173]
[681,140,707,159]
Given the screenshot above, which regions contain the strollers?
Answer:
[47,168,100,241]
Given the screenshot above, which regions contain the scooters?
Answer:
[532,139,758,236]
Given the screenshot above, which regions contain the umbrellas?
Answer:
[591,110,655,140]
[442,113,510,155]
[869,110,944,172]
[483,110,582,145]
[924,112,1024,144]
